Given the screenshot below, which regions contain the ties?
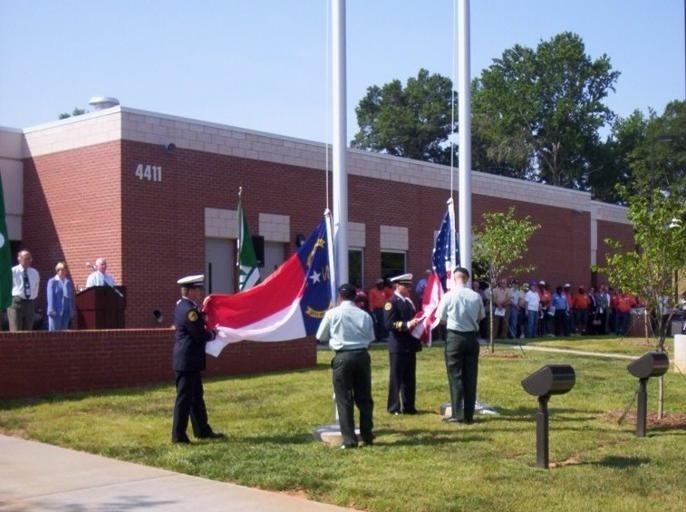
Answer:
[23,271,31,300]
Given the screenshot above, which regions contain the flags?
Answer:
[406,197,461,348]
[202,221,339,358]
[0,177,14,310]
[227,196,264,292]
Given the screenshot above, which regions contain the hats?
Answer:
[177,275,205,287]
[390,273,414,283]
[454,267,470,277]
[538,281,546,286]
[563,283,571,288]
[338,284,353,294]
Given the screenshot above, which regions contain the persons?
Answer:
[526,282,540,340]
[46,261,76,330]
[563,284,576,336]
[538,280,551,338]
[171,274,224,444]
[605,286,611,306]
[599,282,604,288]
[368,279,387,343]
[416,270,433,303]
[659,296,676,339]
[472,281,484,298]
[608,285,617,296]
[493,279,510,340]
[679,292,686,334]
[382,273,422,415]
[315,283,376,451]
[588,287,597,335]
[547,283,552,292]
[596,289,608,336]
[576,285,590,333]
[552,286,569,338]
[352,281,368,311]
[385,280,394,297]
[85,257,115,287]
[509,278,520,340]
[519,282,527,339]
[614,290,635,339]
[434,268,486,424]
[7,251,39,332]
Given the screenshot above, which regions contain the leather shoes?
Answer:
[207,432,223,438]
[443,417,461,422]
[391,410,402,416]
[337,443,358,449]
